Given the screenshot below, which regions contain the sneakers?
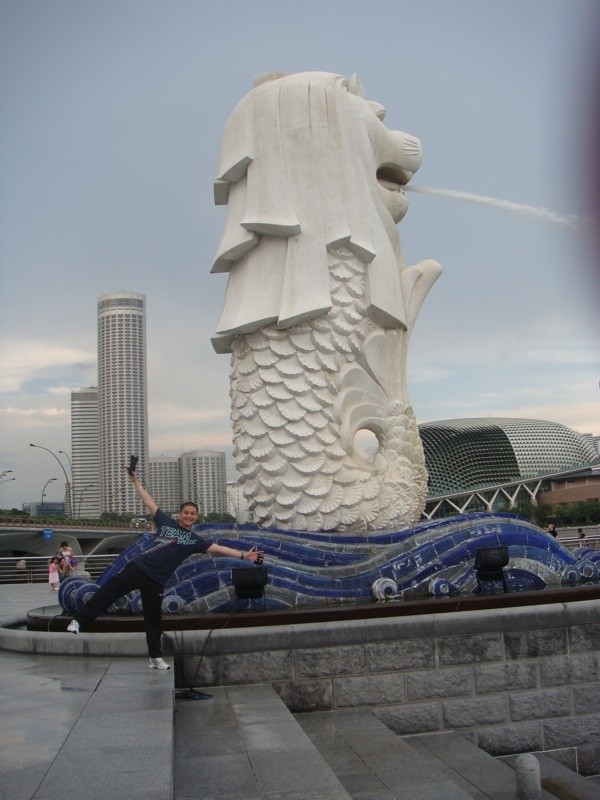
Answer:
[67,619,80,634]
[148,657,170,670]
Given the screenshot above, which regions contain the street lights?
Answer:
[77,484,94,523]
[41,478,58,516]
[59,450,75,524]
[30,443,73,524]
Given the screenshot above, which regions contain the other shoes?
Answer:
[54,587,59,591]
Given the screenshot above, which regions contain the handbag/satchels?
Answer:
[232,567,268,599]
[70,556,78,567]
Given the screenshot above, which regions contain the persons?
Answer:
[49,557,60,591]
[578,527,590,547]
[65,464,264,668]
[548,526,560,541]
[59,539,73,575]
[57,556,74,583]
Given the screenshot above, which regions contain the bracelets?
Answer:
[240,552,244,560]
